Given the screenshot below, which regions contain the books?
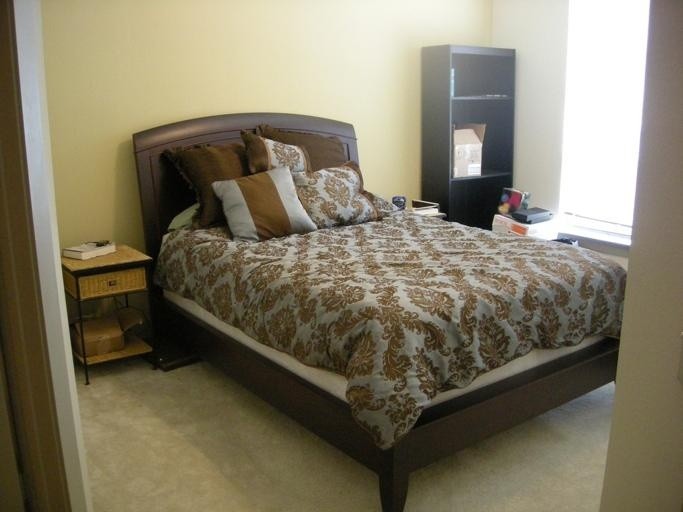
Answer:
[61,240,116,260]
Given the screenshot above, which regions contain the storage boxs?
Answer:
[491,208,560,241]
[71,307,143,357]
[452,123,487,178]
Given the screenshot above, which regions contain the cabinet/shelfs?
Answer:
[421,45,517,231]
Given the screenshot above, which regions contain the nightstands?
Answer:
[61,245,158,385]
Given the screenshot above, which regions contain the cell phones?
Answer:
[97,239,109,246]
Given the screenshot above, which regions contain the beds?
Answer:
[133,114,628,512]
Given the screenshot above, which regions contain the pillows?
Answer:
[163,124,400,244]
